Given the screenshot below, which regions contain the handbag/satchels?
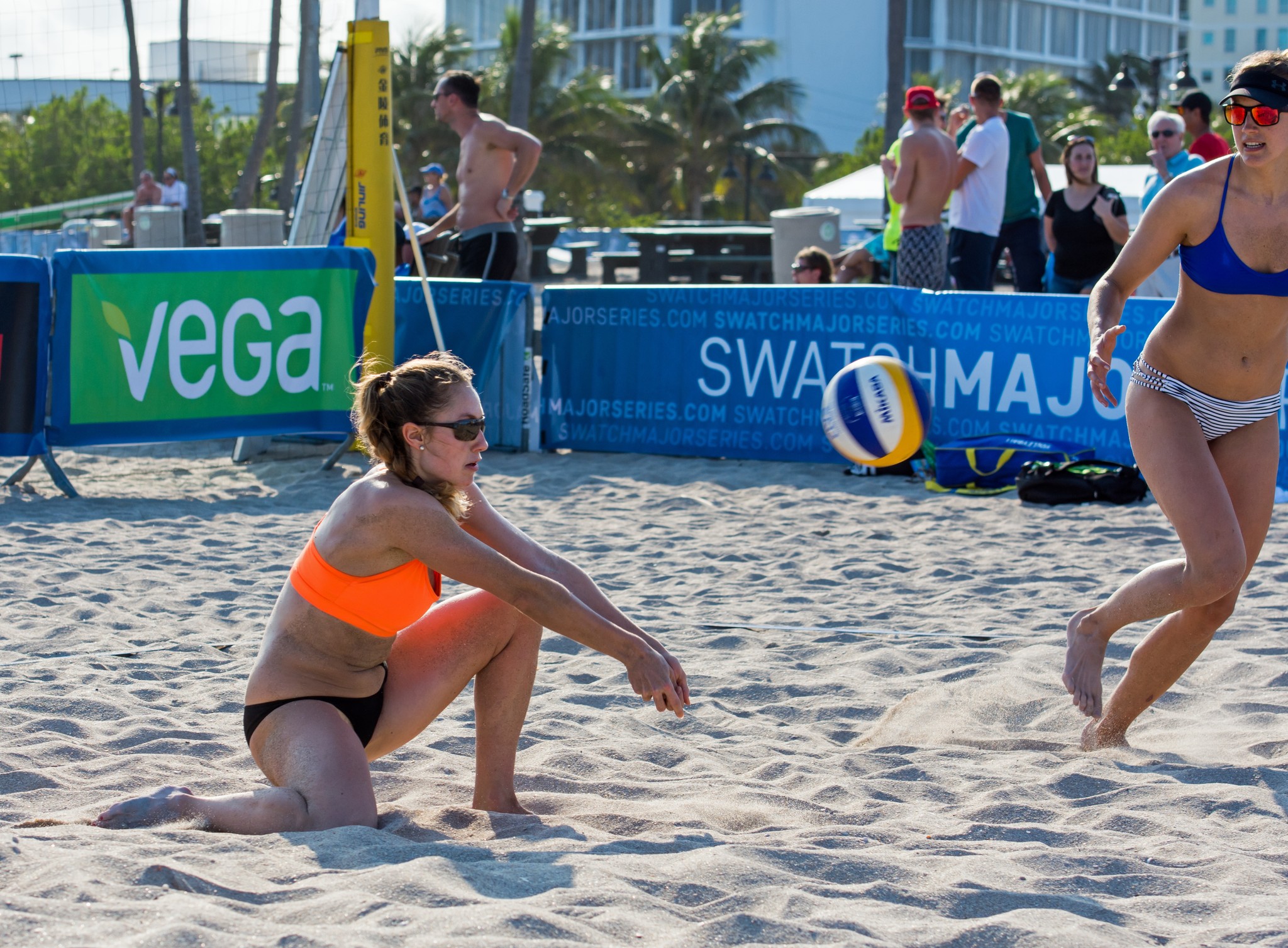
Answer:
[923,433,1095,493]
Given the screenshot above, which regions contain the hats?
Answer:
[1168,91,1212,115]
[905,86,940,109]
[418,163,444,178]
[1218,65,1288,112]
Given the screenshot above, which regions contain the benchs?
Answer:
[563,241,600,279]
[591,249,772,283]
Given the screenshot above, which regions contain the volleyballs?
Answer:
[820,355,931,468]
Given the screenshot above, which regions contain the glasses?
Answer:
[1222,104,1281,127]
[1151,129,1179,139]
[1177,105,1191,116]
[431,92,447,101]
[414,416,486,441]
[791,264,811,274]
[1067,134,1095,146]
[939,115,947,122]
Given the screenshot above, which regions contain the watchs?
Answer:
[500,189,515,204]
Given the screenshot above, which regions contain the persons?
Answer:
[92,350,693,834]
[328,161,455,267]
[838,71,1131,300]
[1134,89,1232,300]
[785,245,839,285]
[416,68,542,282]
[1063,48,1288,756]
[122,166,190,241]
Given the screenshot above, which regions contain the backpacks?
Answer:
[1014,457,1150,508]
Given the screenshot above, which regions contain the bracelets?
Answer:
[1162,171,1173,182]
[1095,206,1108,216]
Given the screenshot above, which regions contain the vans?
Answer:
[995,164,1159,286]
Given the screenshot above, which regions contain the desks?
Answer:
[523,217,573,278]
[620,228,774,284]
